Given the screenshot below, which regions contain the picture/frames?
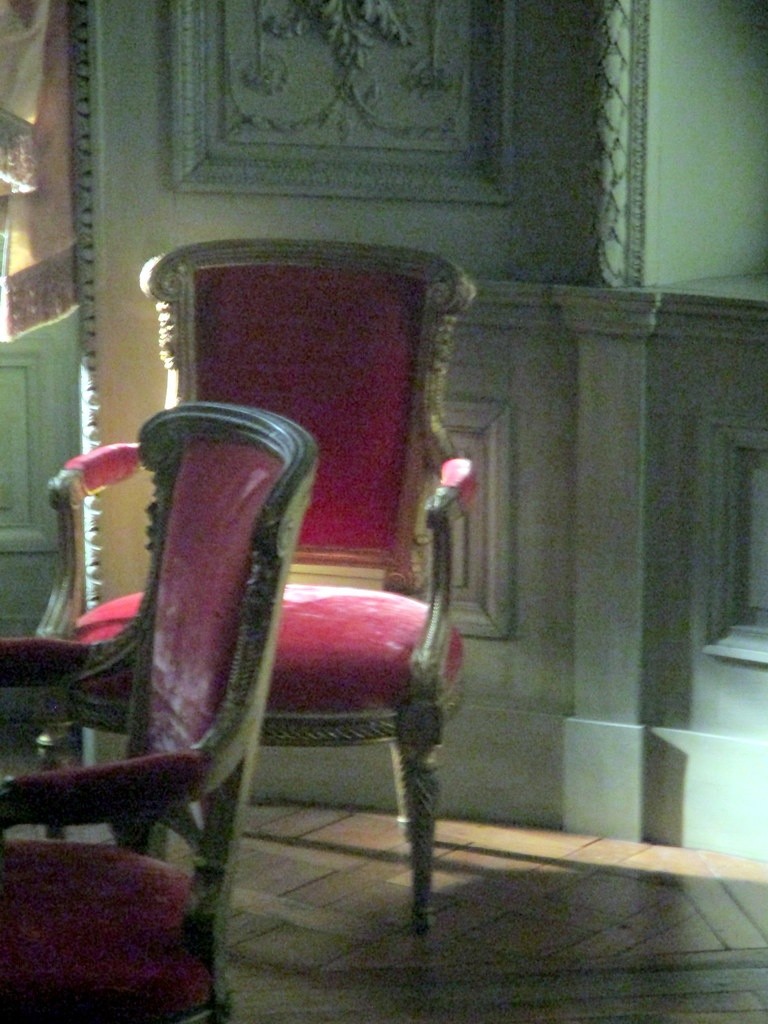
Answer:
[165,0,516,209]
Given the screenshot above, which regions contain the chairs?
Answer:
[38,240,479,935]
[0,402,321,1024]
